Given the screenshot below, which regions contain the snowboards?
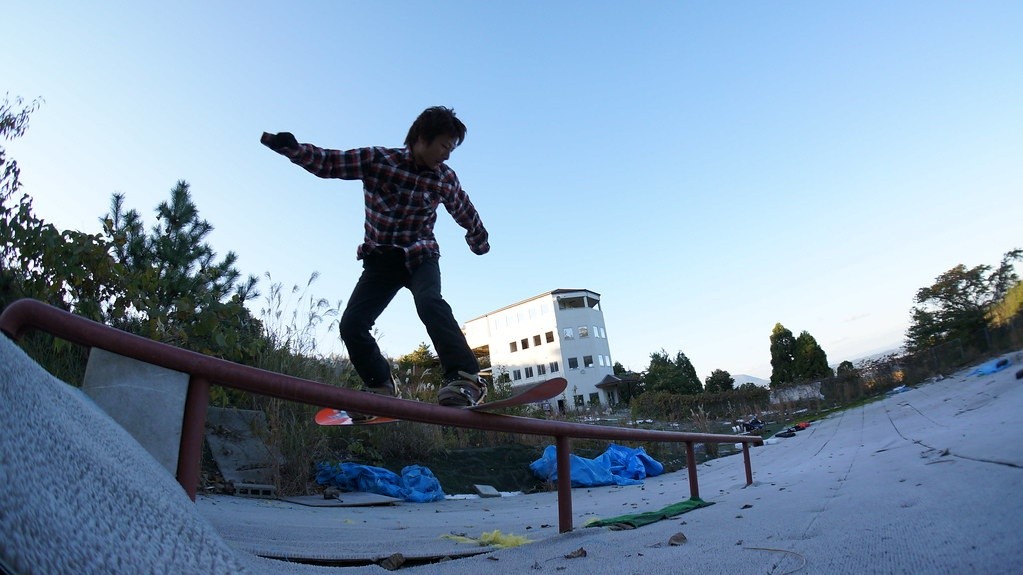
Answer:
[313,376,568,428]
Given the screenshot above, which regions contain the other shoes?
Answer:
[344,374,403,422]
[438,370,484,405]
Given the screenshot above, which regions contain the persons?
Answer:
[260,105,490,420]
